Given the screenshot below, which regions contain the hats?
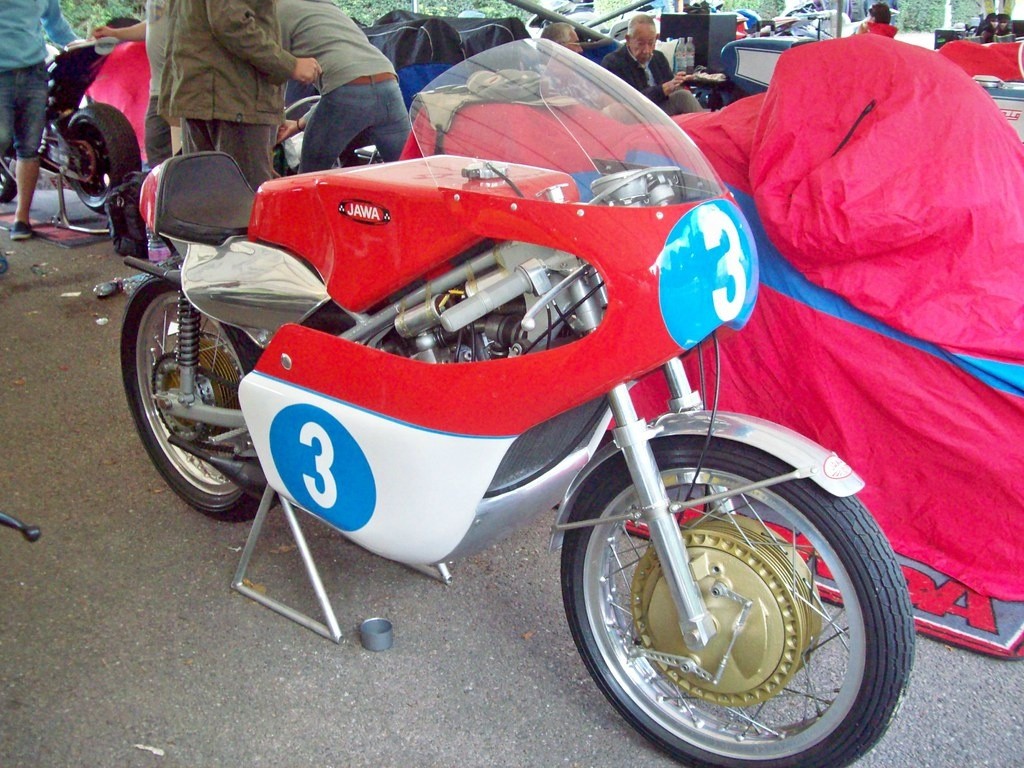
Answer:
[985,13,1010,19]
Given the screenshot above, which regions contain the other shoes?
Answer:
[10,221,33,240]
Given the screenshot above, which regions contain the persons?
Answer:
[976,13,1011,43]
[277,0,412,175]
[92,0,173,170]
[0,0,80,240]
[157,0,322,192]
[851,2,899,39]
[600,14,704,115]
[531,23,647,125]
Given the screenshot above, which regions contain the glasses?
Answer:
[566,42,582,45]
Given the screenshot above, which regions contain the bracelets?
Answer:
[297,119,302,130]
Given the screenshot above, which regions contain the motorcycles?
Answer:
[117,35,919,768]
[0,0,1024,662]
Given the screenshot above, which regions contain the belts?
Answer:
[348,73,396,84]
